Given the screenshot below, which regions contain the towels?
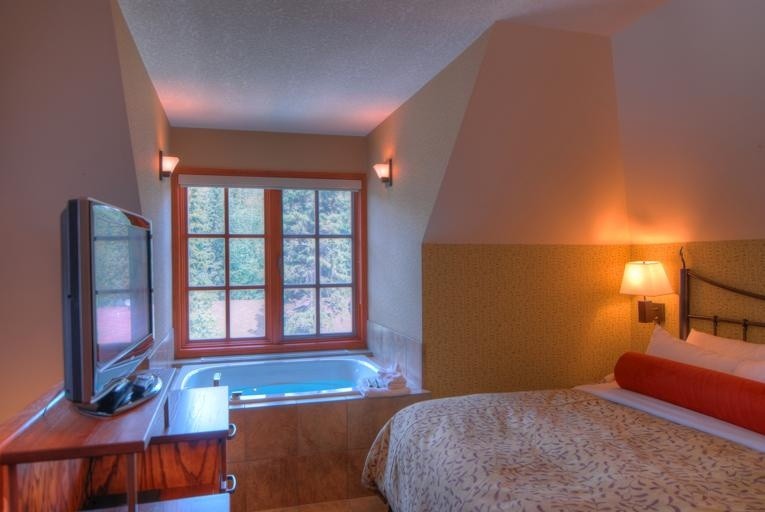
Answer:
[355,368,412,399]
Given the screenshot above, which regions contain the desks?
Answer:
[0,367,237,512]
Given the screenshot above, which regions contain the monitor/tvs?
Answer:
[59,196,163,417]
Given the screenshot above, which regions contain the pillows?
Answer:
[614,323,765,435]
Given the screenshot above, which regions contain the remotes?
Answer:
[132,372,156,393]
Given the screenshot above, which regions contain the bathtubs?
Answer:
[174,358,430,511]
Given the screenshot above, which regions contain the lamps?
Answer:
[159,150,180,180]
[619,260,674,323]
[372,158,392,186]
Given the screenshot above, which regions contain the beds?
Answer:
[360,246,765,511]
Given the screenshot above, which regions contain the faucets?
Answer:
[213,370,222,387]
